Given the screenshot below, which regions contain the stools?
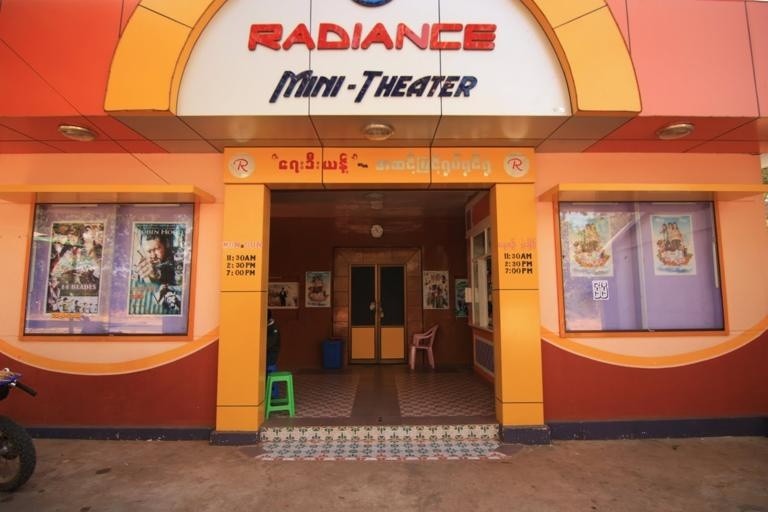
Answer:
[264,372,295,420]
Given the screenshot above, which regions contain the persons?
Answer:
[577,223,600,253]
[134,229,185,315]
[661,222,683,251]
[311,276,327,297]
[426,284,449,309]
[46,223,104,314]
[279,287,287,307]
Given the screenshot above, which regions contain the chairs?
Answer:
[407,324,439,370]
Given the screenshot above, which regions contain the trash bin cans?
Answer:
[322,340,343,369]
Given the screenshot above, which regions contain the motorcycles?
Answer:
[0,367,37,494]
